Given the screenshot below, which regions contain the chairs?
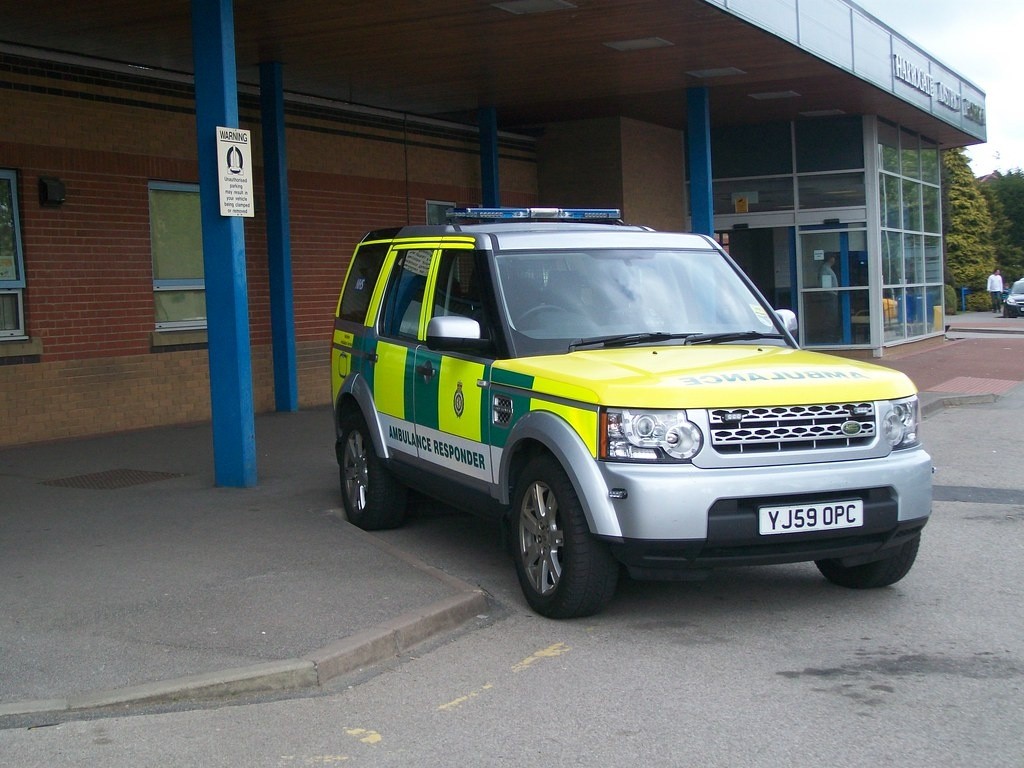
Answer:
[454,266,517,342]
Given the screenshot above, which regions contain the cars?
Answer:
[1002,280,1024,317]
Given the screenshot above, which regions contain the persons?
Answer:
[813,252,838,343]
[987,268,1004,313]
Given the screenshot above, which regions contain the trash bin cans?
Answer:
[897,292,934,334]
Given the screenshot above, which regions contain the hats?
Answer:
[824,252,838,260]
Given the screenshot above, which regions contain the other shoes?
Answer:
[999,309,1001,312]
[991,309,996,313]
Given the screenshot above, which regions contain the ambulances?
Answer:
[330,203,938,622]
